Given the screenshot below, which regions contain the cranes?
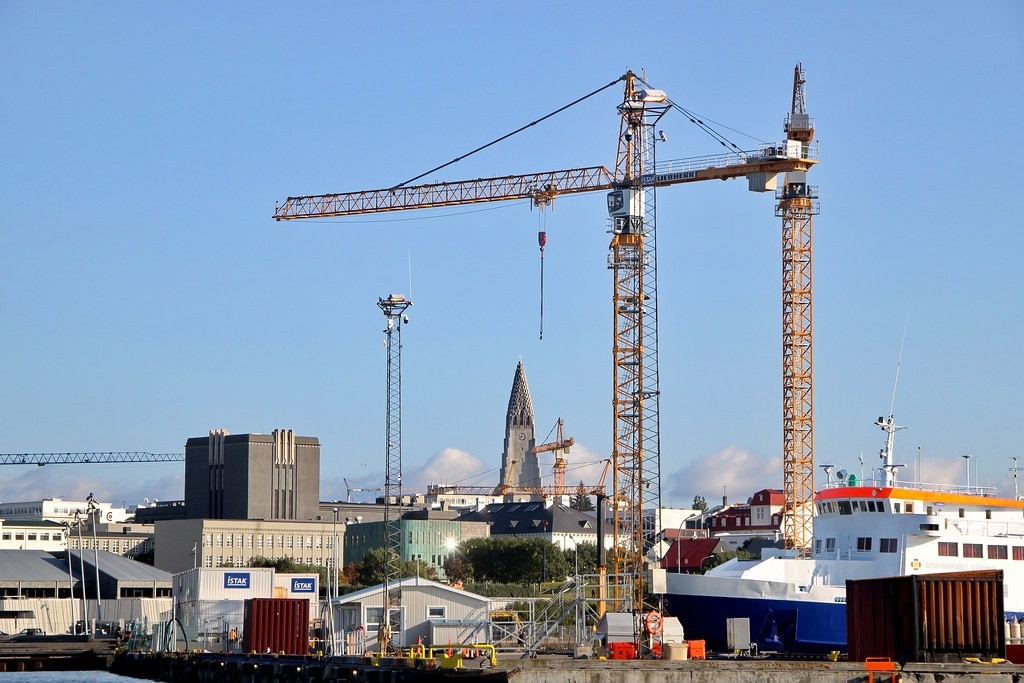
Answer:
[273,66,820,626]
[342,476,414,503]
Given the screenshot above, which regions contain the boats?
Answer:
[666,318,1024,665]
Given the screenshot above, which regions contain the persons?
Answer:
[229,629,236,647]
[591,588,596,598]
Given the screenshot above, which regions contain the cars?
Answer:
[19,628,44,636]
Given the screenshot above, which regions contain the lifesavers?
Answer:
[646,611,663,634]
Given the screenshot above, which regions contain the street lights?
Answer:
[700,555,714,571]
[86,493,103,635]
[569,534,578,646]
[62,520,77,635]
[73,508,88,635]
[678,513,696,574]
